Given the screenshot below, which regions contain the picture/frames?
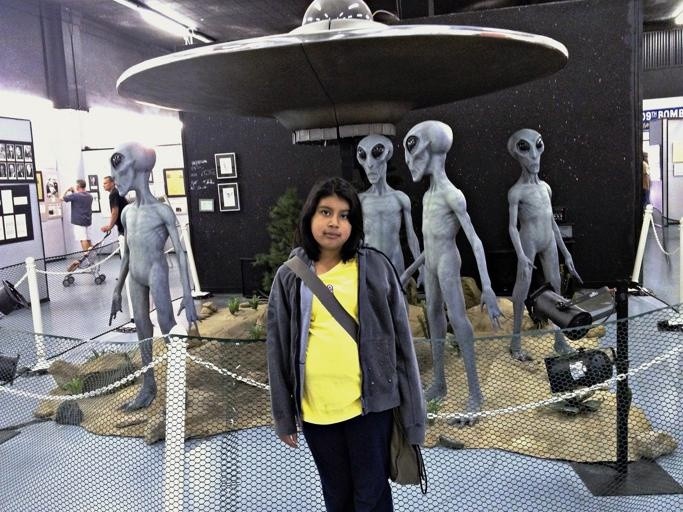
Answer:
[212,152,242,213]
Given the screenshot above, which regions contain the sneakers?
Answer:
[390,407,427,494]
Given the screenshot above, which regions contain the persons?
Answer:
[265,176,429,512]
[506,128,578,365]
[355,134,425,319]
[100,175,129,281]
[642,152,653,214]
[0,144,59,202]
[62,178,94,254]
[399,120,509,430]
[108,140,203,412]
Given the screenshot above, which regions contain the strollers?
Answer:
[63,230,112,287]
